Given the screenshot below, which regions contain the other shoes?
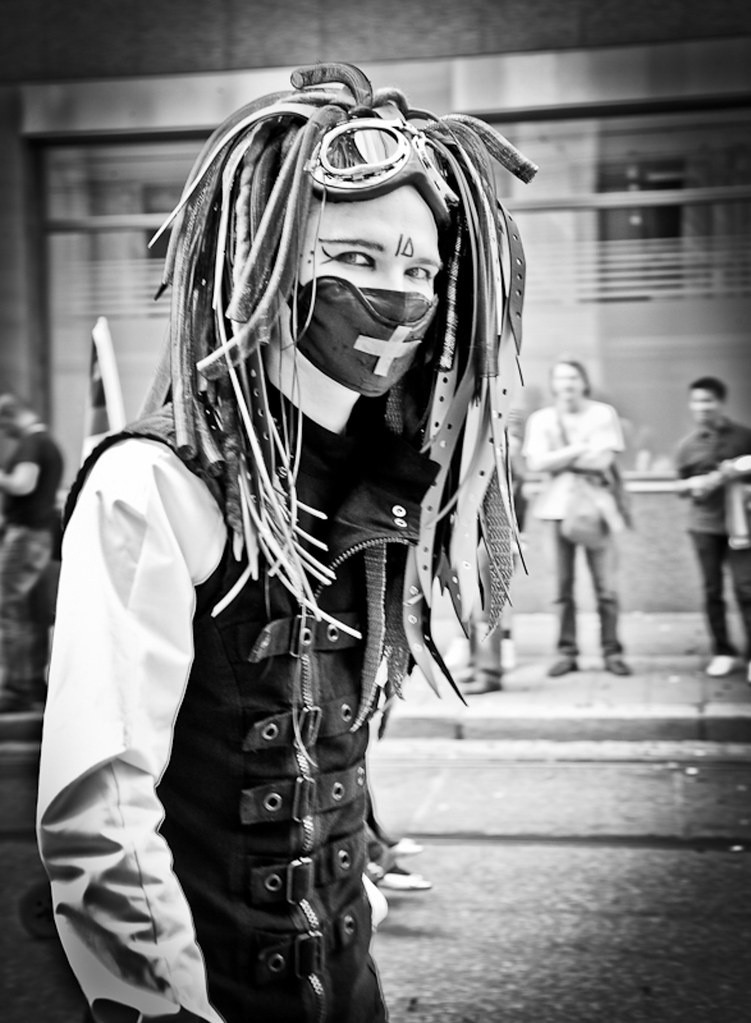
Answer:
[605,660,630,676]
[548,659,577,677]
[705,655,738,676]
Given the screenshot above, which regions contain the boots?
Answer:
[463,618,502,695]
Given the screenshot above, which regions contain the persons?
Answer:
[34,61,540,1023]
[0,393,64,712]
[672,377,751,685]
[522,360,636,678]
[456,400,526,696]
[364,794,431,891]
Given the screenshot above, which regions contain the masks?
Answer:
[287,275,441,396]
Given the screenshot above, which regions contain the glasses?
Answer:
[301,117,454,224]
